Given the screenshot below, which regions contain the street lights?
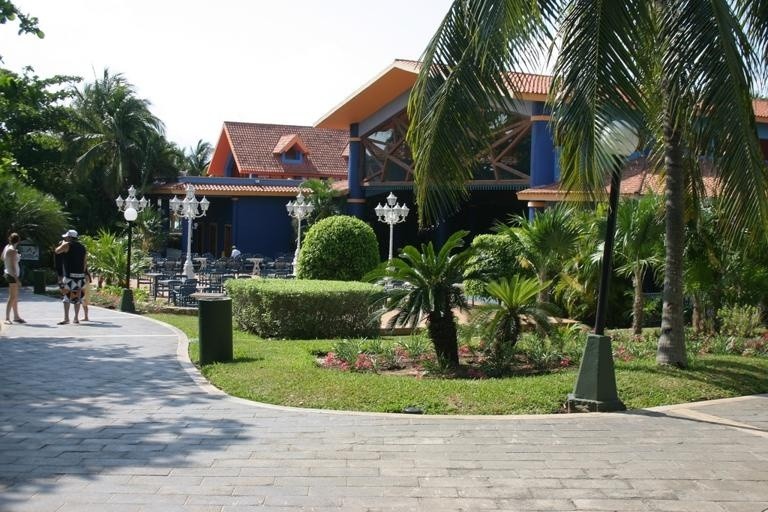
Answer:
[374,192,410,286]
[563,119,640,412]
[115,184,148,312]
[285,191,314,276]
[169,185,210,282]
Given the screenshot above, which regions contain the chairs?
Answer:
[136,247,294,309]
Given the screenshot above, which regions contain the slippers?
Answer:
[4,318,26,324]
[57,318,88,325]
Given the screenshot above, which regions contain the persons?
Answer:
[54,227,87,325]
[229,244,241,262]
[56,238,92,322]
[1,237,27,325]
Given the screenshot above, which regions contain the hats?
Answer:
[61,229,78,238]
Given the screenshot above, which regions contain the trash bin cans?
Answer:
[197,296,233,367]
[33,270,47,293]
